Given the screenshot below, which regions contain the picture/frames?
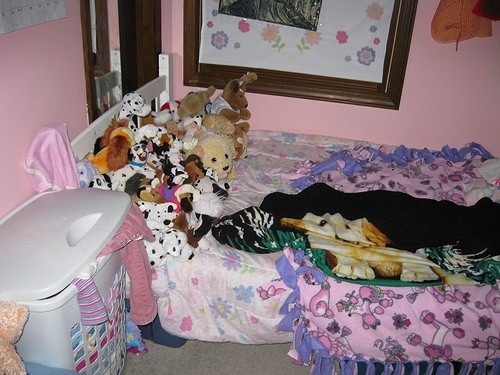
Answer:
[182,0,421,111]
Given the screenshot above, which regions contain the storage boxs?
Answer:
[0,188,131,375]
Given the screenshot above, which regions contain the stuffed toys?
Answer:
[88,71,261,266]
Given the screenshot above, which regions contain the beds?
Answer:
[69,52,500,375]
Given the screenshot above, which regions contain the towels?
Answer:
[122,240,157,325]
